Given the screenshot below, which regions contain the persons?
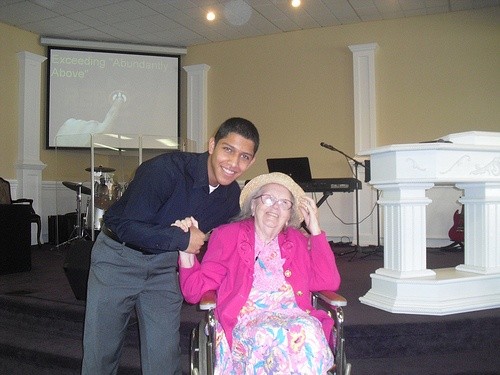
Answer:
[170,172,341,375]
[81,117,259,375]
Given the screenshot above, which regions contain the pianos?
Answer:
[242,178,361,235]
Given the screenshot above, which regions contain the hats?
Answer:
[238,172,311,226]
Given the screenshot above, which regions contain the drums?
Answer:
[91,177,123,210]
[83,197,106,231]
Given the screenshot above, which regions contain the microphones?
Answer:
[320,142,336,151]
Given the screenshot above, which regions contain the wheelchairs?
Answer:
[191,221,352,375]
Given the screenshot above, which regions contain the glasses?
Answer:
[253,194,293,210]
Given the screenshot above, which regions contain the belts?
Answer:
[101,224,153,255]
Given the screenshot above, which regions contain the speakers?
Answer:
[48,215,79,245]
[64,240,94,300]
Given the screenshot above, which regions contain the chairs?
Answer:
[0,177,42,247]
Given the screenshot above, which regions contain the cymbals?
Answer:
[84,167,114,172]
[62,181,92,196]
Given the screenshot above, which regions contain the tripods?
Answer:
[332,146,385,262]
[48,186,90,252]
